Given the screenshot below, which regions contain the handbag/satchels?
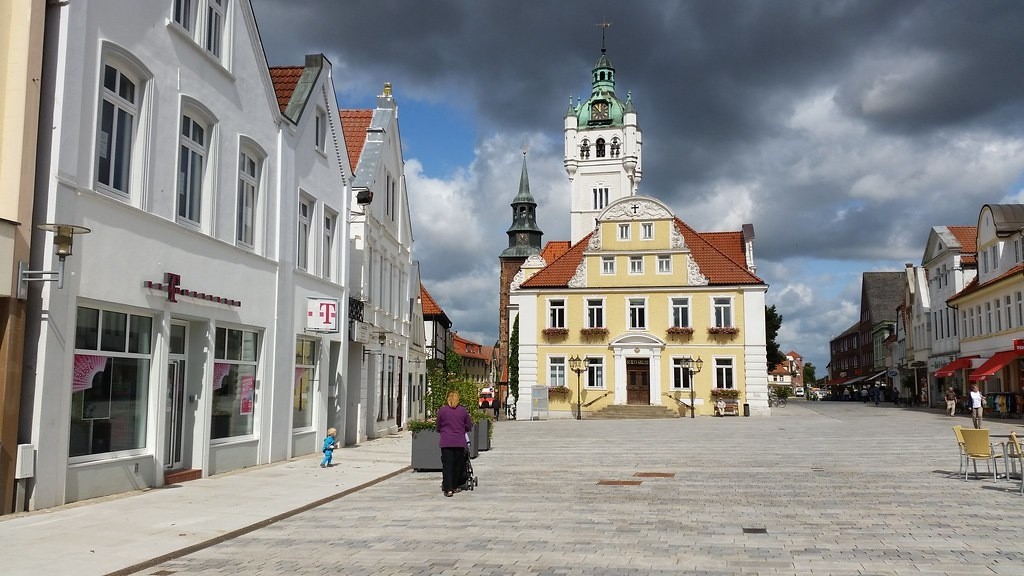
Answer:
[981,395,987,408]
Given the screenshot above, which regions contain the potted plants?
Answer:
[405,347,495,472]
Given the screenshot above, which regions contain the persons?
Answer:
[320,428,336,468]
[969,384,984,429]
[945,387,956,416]
[717,398,726,416]
[492,395,501,420]
[437,392,473,496]
[482,397,488,407]
[810,389,818,400]
[837,387,875,403]
[873,387,880,407]
[893,387,899,405]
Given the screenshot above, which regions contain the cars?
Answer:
[796,390,804,397]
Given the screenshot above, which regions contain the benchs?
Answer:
[714,403,738,417]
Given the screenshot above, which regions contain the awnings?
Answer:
[934,358,970,377]
[968,349,1024,381]
[823,378,854,385]
[843,377,865,384]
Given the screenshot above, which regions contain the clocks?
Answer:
[590,101,609,120]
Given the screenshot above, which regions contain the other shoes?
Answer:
[453,487,461,493]
[320,463,326,468]
[327,464,333,467]
[444,491,453,496]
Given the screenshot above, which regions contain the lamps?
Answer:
[373,331,393,346]
[17,223,91,299]
[409,356,425,369]
[351,190,374,223]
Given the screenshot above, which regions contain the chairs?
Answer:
[960,428,1007,482]
[952,425,999,478]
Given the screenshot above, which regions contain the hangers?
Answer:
[986,392,1018,397]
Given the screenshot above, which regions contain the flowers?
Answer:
[548,385,570,394]
[710,387,742,398]
[665,325,694,336]
[580,327,609,335]
[541,327,570,336]
[708,324,740,336]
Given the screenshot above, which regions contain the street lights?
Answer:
[680,354,704,418]
[568,354,589,419]
